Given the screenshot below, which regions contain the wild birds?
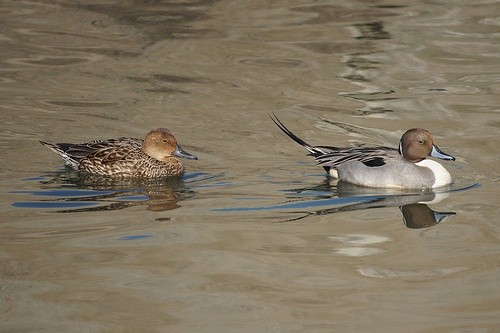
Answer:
[40,127,199,180]
[268,111,456,190]
